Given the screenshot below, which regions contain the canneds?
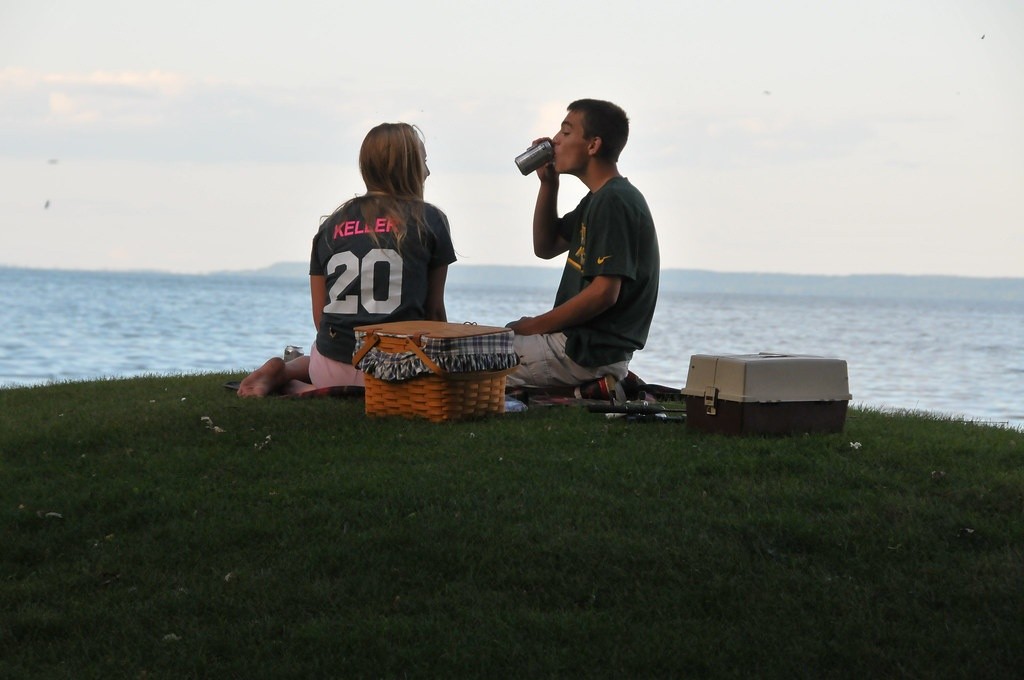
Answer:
[515,141,555,176]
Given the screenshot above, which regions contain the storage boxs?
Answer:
[680,351,853,437]
[350,319,522,423]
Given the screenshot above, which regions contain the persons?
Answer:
[236,123,458,398]
[503,98,661,399]
